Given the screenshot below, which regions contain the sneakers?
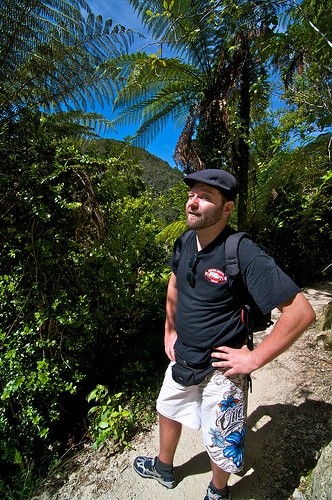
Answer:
[134,456,177,488]
[204,484,232,500]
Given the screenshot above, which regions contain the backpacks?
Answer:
[171,229,273,333]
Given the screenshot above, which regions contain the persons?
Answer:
[131,168,314,500]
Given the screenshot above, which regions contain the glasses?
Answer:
[187,251,199,288]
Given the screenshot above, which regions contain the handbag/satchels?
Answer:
[171,339,217,386]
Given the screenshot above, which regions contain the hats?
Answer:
[183,168,239,200]
